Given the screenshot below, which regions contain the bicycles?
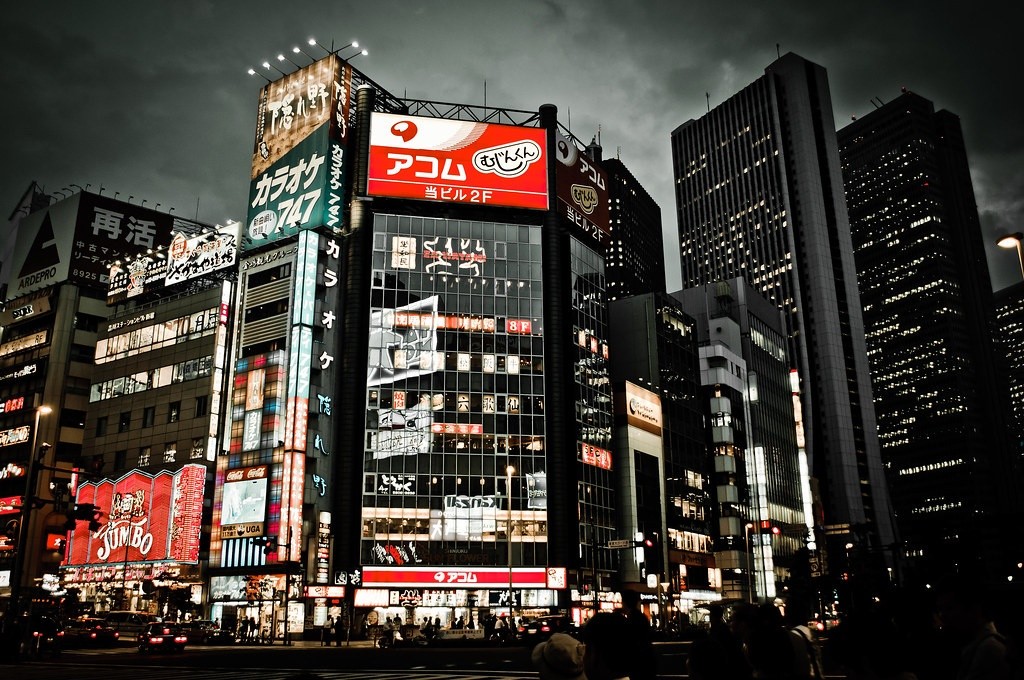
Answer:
[414,630,443,648]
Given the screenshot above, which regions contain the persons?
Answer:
[239,617,256,645]
[479,563,1011,680]
[322,614,344,647]
[360,614,475,648]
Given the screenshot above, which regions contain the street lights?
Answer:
[744,523,754,607]
[505,464,516,644]
[119,492,134,610]
[3,404,54,661]
[846,542,854,607]
[652,532,664,639]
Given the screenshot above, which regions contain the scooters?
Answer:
[378,626,404,649]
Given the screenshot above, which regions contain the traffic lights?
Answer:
[255,538,272,548]
[635,539,655,548]
[578,582,589,594]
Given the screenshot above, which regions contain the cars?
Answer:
[1,608,236,661]
[517,615,569,648]
